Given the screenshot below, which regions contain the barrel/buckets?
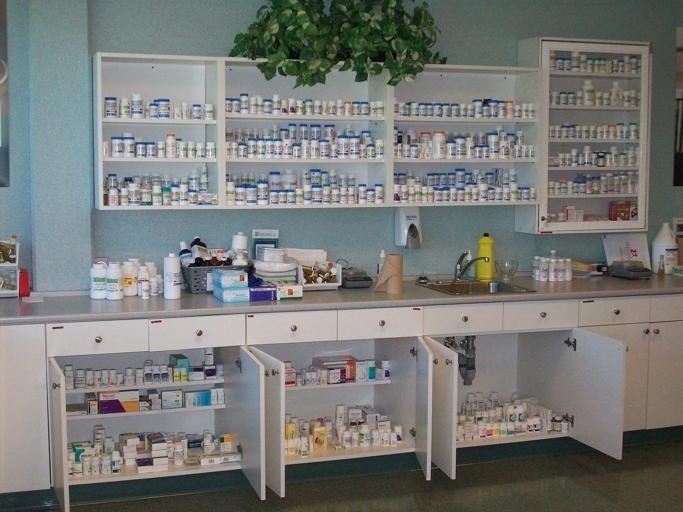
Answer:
[651,223,679,275]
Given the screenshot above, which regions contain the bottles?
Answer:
[224,92,384,204]
[286,404,404,457]
[179,231,250,267]
[205,349,215,367]
[547,50,642,221]
[376,249,386,275]
[89,254,185,300]
[531,250,574,282]
[64,427,120,481]
[283,361,329,385]
[657,254,666,279]
[366,360,390,383]
[63,365,187,389]
[102,92,218,206]
[394,95,537,202]
[455,393,572,439]
[163,428,214,465]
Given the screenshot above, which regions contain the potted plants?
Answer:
[228,0,448,88]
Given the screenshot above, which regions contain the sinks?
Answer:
[415,278,537,296]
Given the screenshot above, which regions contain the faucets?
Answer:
[454,250,489,280]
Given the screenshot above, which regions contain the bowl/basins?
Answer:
[494,261,518,281]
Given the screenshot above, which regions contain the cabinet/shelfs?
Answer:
[1,323,48,511]
[422,298,628,482]
[246,306,434,499]
[578,292,683,451]
[46,314,265,512]
[514,35,655,235]
[93,51,539,212]
